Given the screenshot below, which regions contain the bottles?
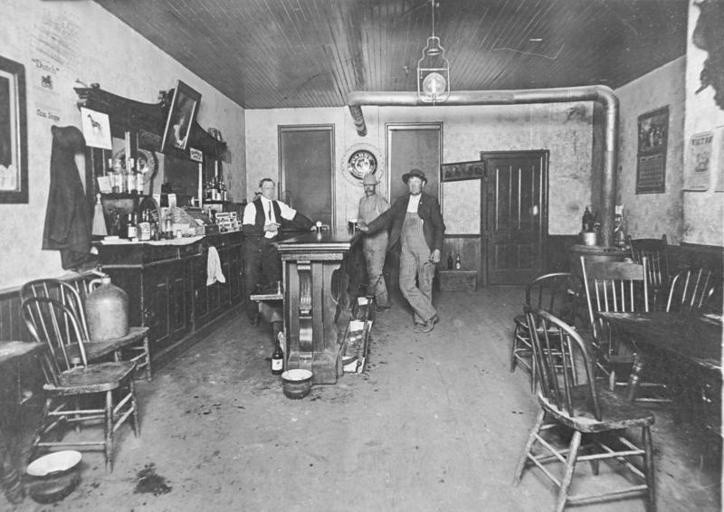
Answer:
[101,155,173,241]
[272,339,283,374]
[205,175,229,201]
[446,251,462,271]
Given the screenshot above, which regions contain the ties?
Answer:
[269,201,271,221]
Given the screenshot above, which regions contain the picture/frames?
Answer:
[159,78,205,165]
[0,54,29,204]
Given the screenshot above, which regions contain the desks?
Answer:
[0,338,51,504]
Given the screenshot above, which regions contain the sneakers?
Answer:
[375,305,392,311]
[413,313,439,332]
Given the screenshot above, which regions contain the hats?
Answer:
[360,173,382,186]
[402,169,427,185]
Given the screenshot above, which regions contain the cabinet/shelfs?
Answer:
[87,230,245,370]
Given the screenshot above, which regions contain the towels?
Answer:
[205,246,226,287]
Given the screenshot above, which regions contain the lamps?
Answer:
[414,0,450,103]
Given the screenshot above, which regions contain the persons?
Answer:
[172,108,188,148]
[242,177,330,327]
[358,170,447,331]
[345,174,394,312]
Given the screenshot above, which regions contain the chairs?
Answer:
[16,278,152,475]
